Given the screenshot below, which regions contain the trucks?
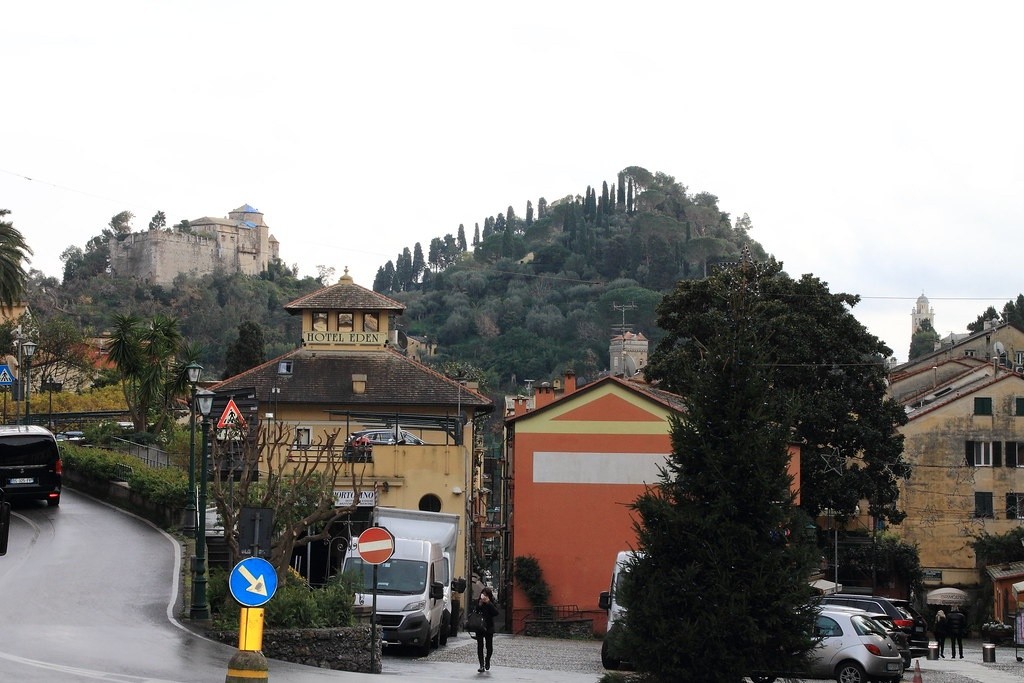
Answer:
[370,505,468,647]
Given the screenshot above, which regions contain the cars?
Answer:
[746,607,904,683]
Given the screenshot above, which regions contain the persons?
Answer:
[933,609,947,659]
[947,602,966,659]
[470,586,499,673]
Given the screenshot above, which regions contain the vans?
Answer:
[596,550,654,670]
[1,424,63,506]
[340,536,445,656]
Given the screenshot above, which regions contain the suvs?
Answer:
[810,594,932,658]
[342,430,428,463]
[815,604,910,668]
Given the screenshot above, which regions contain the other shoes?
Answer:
[940,654,945,658]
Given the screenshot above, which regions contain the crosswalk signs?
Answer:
[0,364,16,386]
[216,400,248,429]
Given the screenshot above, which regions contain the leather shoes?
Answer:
[960,655,964,659]
[952,655,955,658]
[478,663,485,672]
[485,657,490,670]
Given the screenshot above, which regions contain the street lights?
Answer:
[182,361,202,538]
[189,389,217,617]
[20,341,37,424]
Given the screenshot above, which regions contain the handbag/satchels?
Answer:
[466,604,487,640]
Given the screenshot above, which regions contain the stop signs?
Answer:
[358,527,393,566]
